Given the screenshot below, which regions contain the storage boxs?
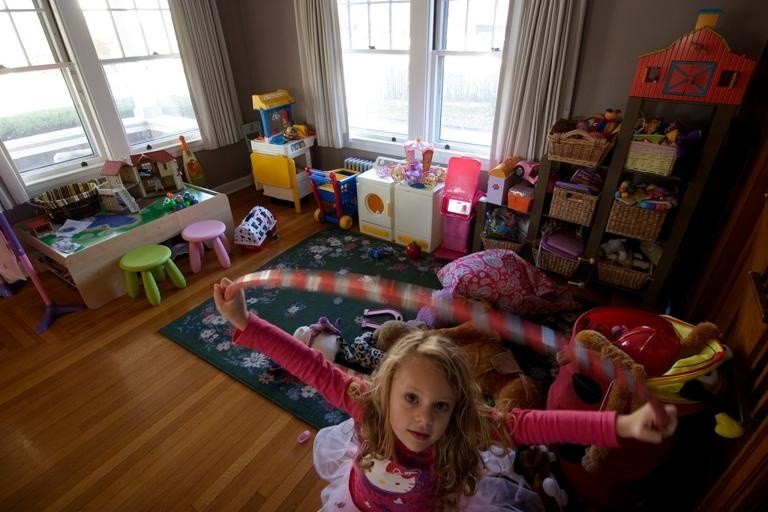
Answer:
[480,220,525,253]
[534,113,609,278]
[598,119,683,289]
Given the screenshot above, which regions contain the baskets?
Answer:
[548,129,616,168]
[624,140,677,177]
[596,262,649,290]
[549,186,600,227]
[532,240,580,277]
[480,231,524,253]
[34,182,101,223]
[605,200,667,243]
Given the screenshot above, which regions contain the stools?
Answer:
[118,244,187,306]
[180,217,234,272]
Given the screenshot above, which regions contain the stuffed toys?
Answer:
[295,314,426,370]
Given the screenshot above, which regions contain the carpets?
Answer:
[157,222,610,432]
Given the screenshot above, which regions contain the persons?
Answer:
[212,278,678,512]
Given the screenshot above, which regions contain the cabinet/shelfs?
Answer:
[526,96,738,313]
